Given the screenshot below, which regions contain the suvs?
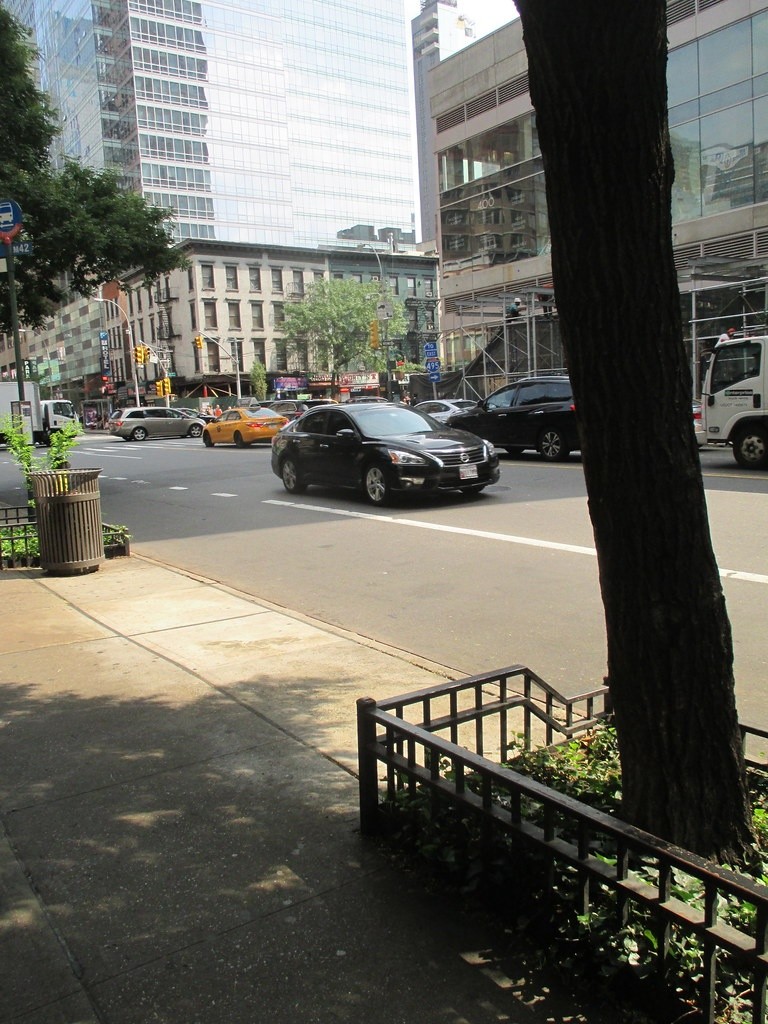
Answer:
[268,400,331,422]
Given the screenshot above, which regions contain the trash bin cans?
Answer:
[27,468,105,574]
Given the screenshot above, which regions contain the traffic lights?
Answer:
[155,380,163,397]
[369,320,378,349]
[163,378,171,395]
[195,335,203,350]
[135,347,143,364]
[143,347,150,365]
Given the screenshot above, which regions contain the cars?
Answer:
[202,407,289,448]
[345,396,389,403]
[270,401,501,504]
[444,375,582,460]
[413,398,478,424]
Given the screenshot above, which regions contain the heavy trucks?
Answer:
[0,381,77,448]
[698,327,768,473]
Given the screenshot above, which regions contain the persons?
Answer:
[195,405,237,417]
[90,412,109,429]
[715,328,735,347]
[538,284,553,318]
[509,298,526,317]
[402,393,421,406]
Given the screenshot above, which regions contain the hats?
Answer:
[727,328,735,334]
[514,298,522,302]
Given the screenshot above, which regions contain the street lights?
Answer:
[18,329,54,400]
[93,297,140,407]
[357,243,392,402]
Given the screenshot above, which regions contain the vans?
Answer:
[106,406,207,441]
